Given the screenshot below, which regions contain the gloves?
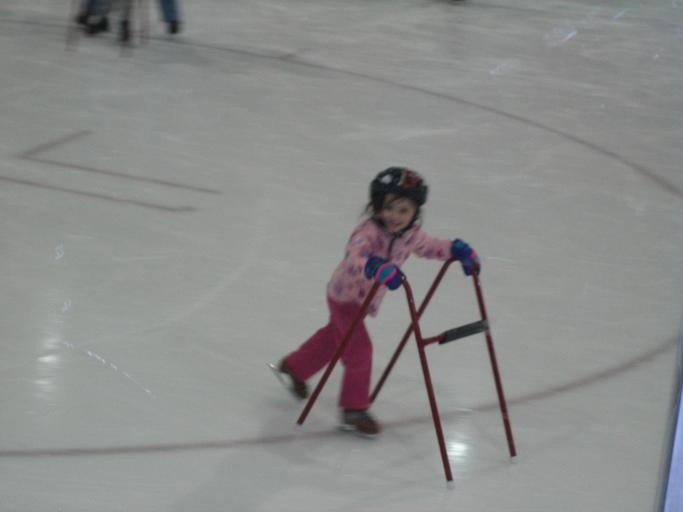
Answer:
[364,256,404,290]
[451,238,480,275]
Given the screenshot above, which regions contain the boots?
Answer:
[341,408,379,433]
[274,360,308,399]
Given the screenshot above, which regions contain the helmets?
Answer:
[369,166,428,210]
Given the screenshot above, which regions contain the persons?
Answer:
[80,1,180,34]
[277,167,479,435]
